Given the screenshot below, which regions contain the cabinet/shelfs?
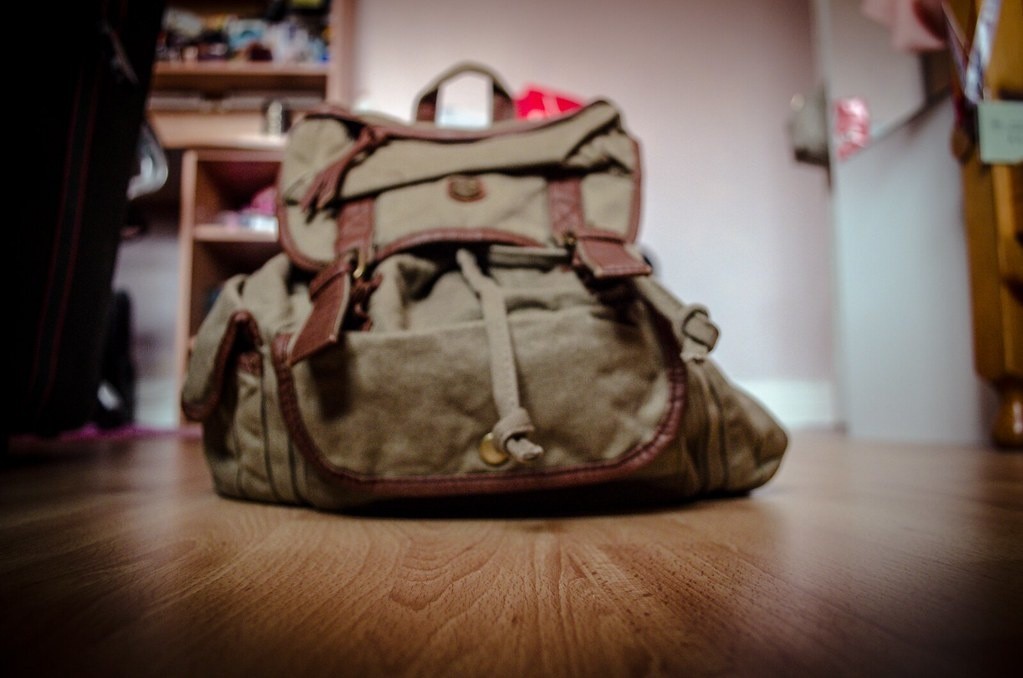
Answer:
[960,0,1023,449]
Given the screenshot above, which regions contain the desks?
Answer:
[149,0,358,460]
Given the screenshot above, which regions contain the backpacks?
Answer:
[180,59,792,516]
